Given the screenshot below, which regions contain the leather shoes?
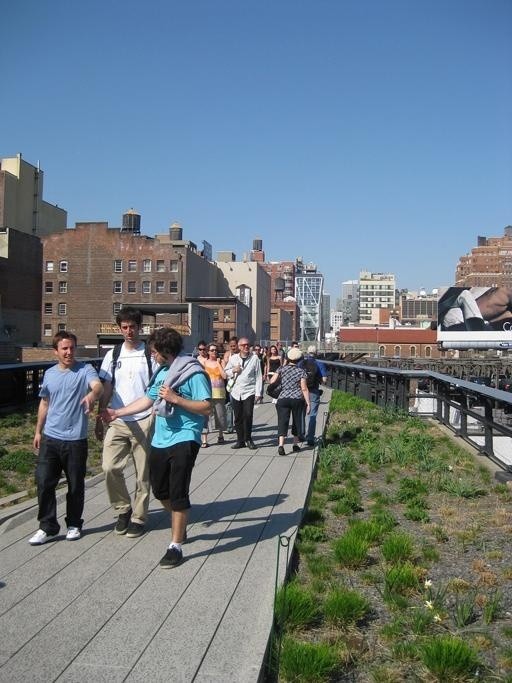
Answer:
[230,434,316,455]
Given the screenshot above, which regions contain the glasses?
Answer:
[209,349,216,352]
[199,348,206,351]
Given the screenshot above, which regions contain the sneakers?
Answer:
[27,507,188,569]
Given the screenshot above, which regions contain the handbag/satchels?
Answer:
[226,373,238,392]
[266,377,282,399]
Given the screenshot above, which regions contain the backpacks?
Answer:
[302,359,319,389]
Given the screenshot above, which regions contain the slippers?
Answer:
[217,436,224,443]
[201,443,207,448]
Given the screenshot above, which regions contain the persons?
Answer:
[194,336,328,457]
[29,329,104,546]
[97,327,212,569]
[93,307,160,539]
